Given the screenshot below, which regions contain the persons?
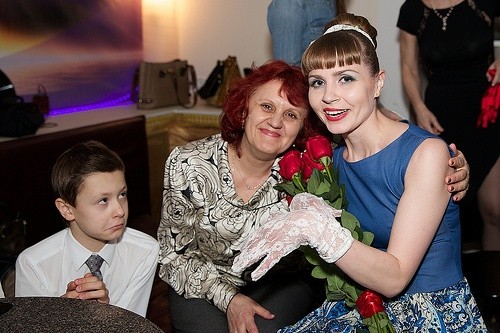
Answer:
[232,14,489,333]
[156,58,471,333]
[395,0,500,251]
[266,0,337,70]
[472,158,500,320]
[15,139,160,319]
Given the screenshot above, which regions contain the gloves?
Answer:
[288,192,343,217]
[230,209,355,282]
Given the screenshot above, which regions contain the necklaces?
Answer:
[430,0,456,30]
[232,148,268,190]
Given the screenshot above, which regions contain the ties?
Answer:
[84,255,105,284]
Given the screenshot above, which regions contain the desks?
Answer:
[1,298,166,333]
[0,75,234,268]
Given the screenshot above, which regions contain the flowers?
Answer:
[277,135,398,333]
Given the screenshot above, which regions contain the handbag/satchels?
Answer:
[197,56,244,111]
[1,95,45,138]
[134,59,198,113]
